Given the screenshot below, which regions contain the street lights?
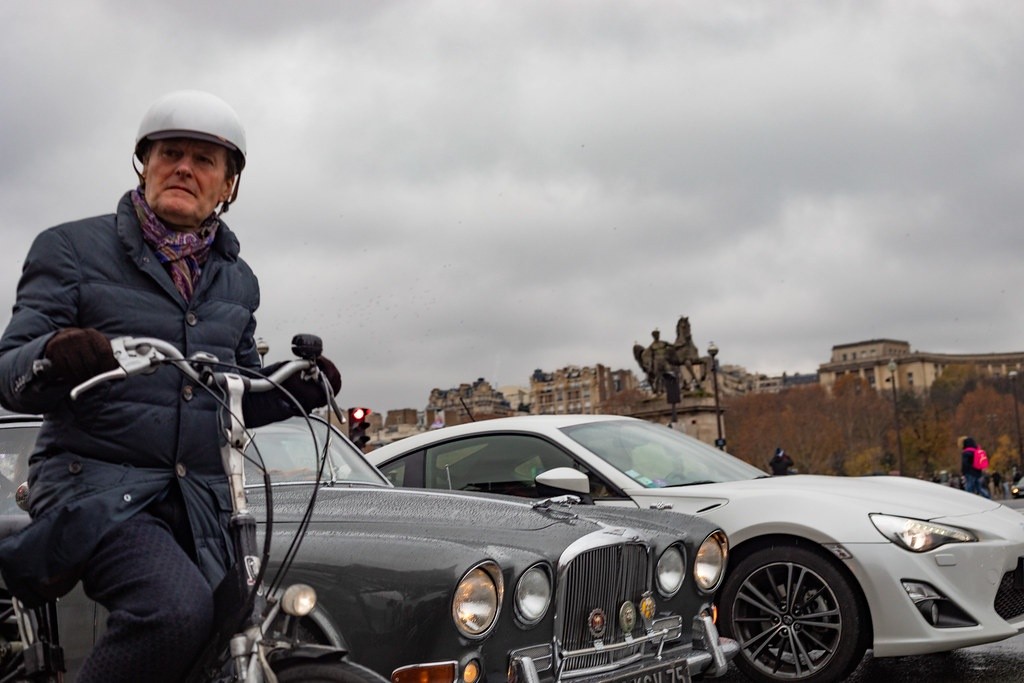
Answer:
[707,339,731,447]
[887,355,909,474]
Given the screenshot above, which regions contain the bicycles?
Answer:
[0,328,389,682]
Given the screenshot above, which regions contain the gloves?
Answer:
[292,357,344,408]
[42,328,112,375]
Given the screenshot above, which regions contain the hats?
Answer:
[774,447,784,457]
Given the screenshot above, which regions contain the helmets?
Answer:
[134,87,248,174]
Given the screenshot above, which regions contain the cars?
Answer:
[0,409,740,683]
[363,409,1024,683]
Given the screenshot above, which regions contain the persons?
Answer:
[0,90,340,683]
[957,436,989,497]
[931,465,1022,498]
[645,331,687,375]
[770,448,794,476]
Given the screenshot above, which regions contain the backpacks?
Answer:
[962,444,989,470]
